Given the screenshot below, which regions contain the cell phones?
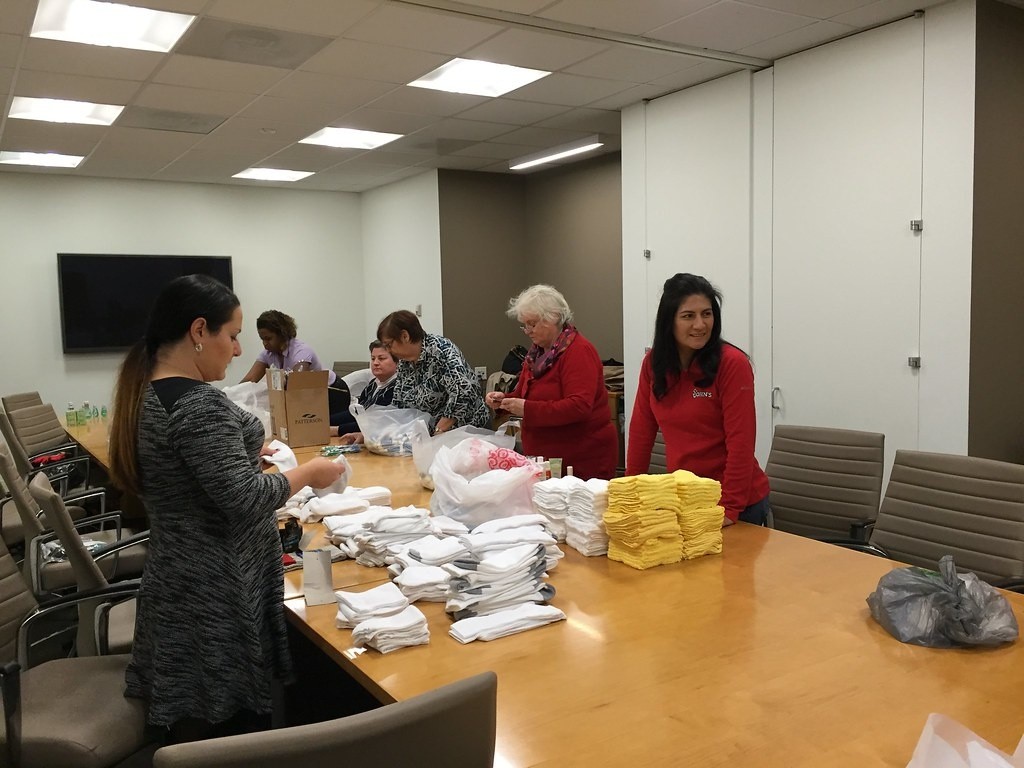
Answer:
[492,398,502,404]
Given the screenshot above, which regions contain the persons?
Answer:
[239,310,490,446]
[106,274,346,736]
[624,273,770,528]
[486,285,618,480]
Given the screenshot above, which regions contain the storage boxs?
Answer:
[265,367,331,449]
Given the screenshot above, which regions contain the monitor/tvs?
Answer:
[57,253,233,354]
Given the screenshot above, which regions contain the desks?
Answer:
[59,412,1024,768]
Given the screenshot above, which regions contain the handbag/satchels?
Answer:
[429,438,544,530]
[30,451,86,491]
[412,419,521,490]
[866,555,1019,649]
[349,402,432,457]
[222,381,273,439]
[502,344,528,392]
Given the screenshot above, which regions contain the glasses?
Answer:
[383,338,396,351]
[519,316,544,332]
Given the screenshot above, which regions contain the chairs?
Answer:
[810,449,1024,594]
[763,424,885,542]
[0,391,497,768]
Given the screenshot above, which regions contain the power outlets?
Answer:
[475,367,488,382]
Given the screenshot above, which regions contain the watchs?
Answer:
[435,426,442,432]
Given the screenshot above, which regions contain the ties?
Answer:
[277,353,284,369]
